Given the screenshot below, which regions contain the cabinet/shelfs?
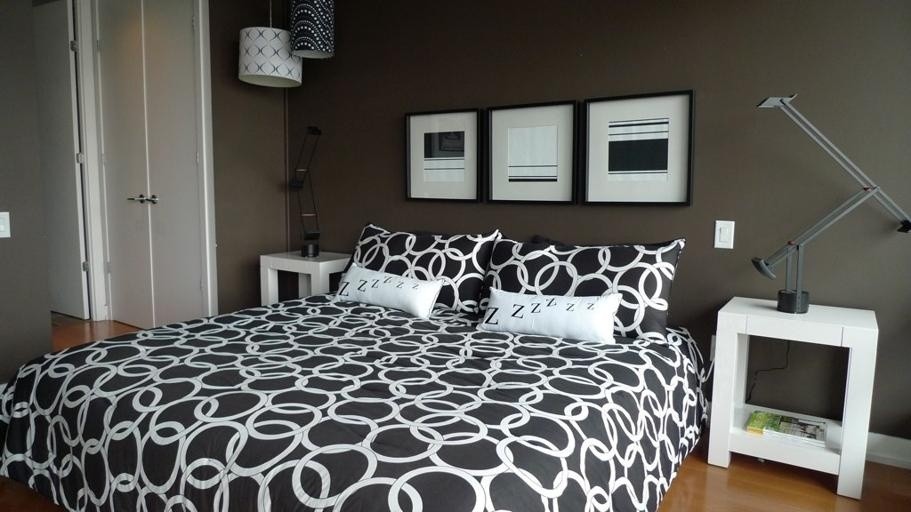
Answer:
[94,0,154,330]
[142,0,218,329]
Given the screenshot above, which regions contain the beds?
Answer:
[1,292,705,512]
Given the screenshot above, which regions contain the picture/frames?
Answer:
[585,88,694,206]
[487,98,577,204]
[405,107,482,203]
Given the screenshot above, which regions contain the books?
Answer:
[744,410,829,448]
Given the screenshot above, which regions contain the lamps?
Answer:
[238,26,303,89]
[290,123,334,255]
[751,90,910,315]
[288,0,335,61]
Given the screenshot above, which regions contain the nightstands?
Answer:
[706,294,880,499]
[259,250,354,306]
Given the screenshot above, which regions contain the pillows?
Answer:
[338,263,444,320]
[478,290,620,350]
[477,235,684,337]
[348,223,498,320]
